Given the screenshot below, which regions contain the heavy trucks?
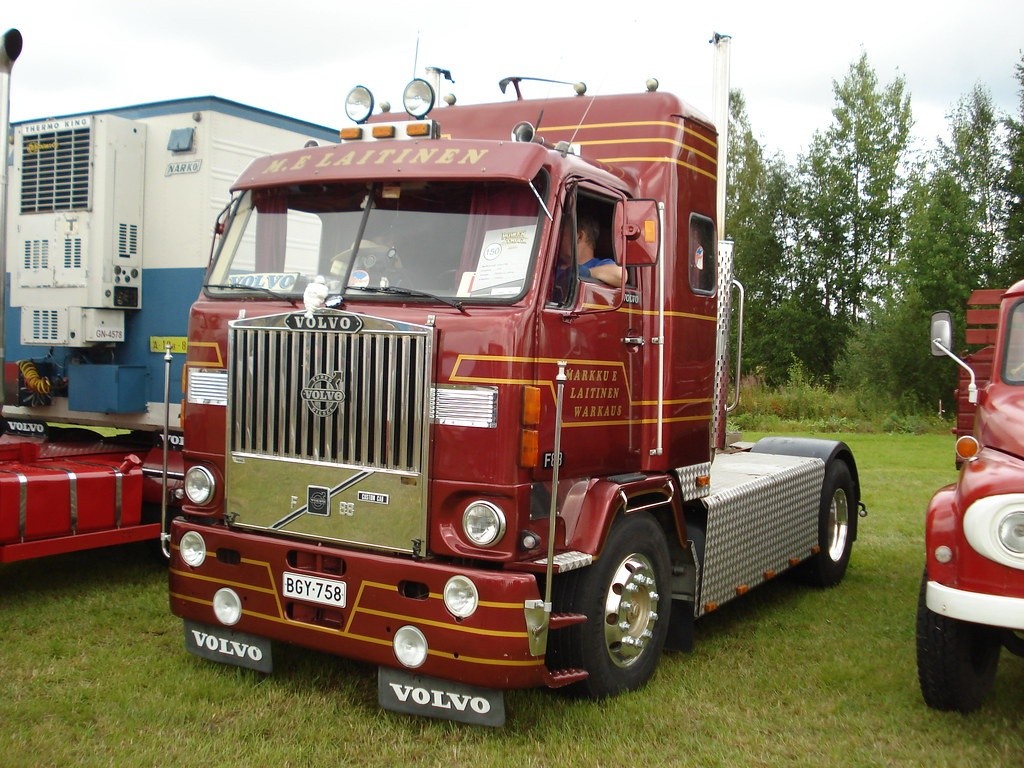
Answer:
[159,31,867,734]
[0,28,343,569]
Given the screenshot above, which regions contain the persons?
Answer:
[554,208,628,287]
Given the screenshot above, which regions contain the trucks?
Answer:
[915,278,1024,712]
